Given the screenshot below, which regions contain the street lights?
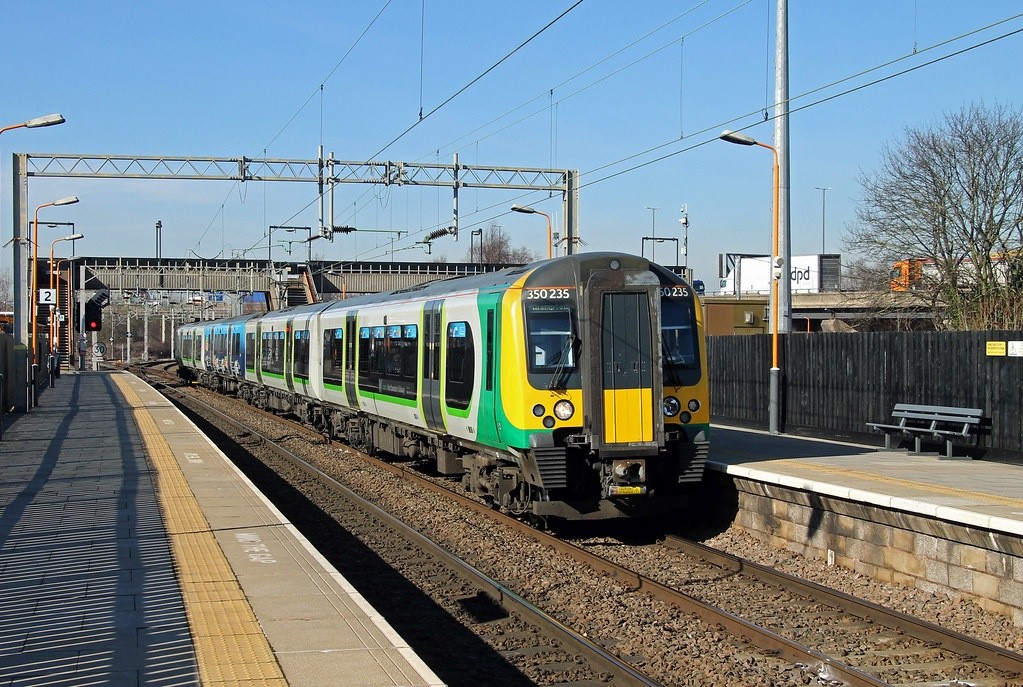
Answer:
[49,233,85,356]
[32,195,79,365]
[719,131,783,434]
[510,204,552,260]
[55,255,82,354]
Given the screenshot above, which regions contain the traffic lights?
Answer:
[86,302,101,332]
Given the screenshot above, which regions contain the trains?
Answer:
[170,246,716,531]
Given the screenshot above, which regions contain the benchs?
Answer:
[865,403,983,462]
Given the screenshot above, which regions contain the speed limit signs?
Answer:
[92,342,107,356]
